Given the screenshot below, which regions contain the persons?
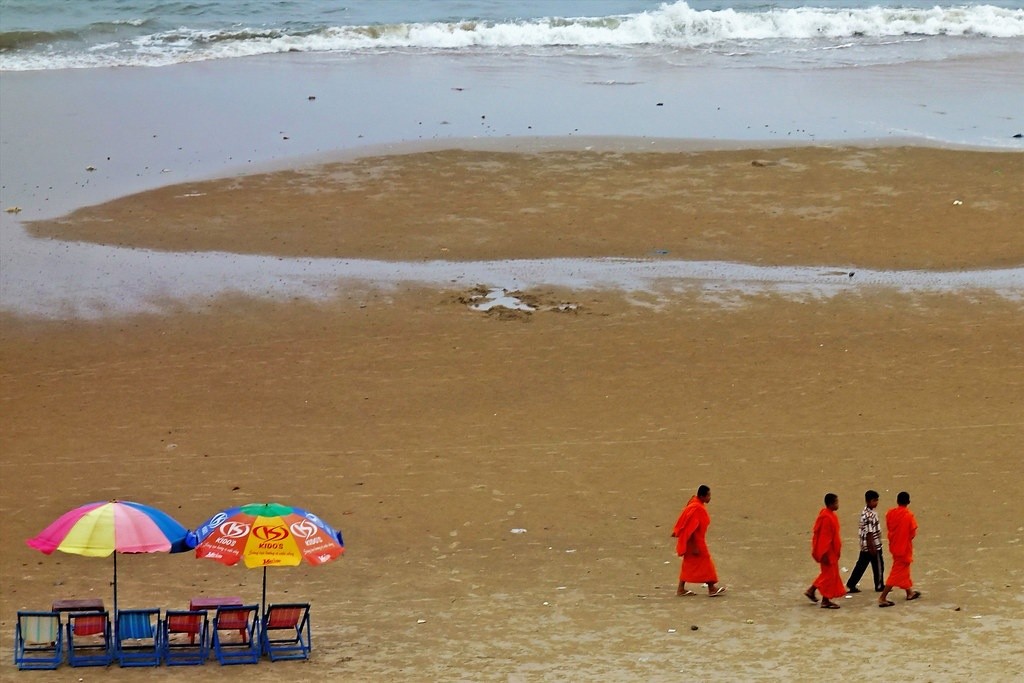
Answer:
[803,493,848,609]
[672,484,724,597]
[878,491,920,607]
[847,490,893,593]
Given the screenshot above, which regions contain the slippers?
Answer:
[877,586,893,592]
[676,591,696,597]
[804,590,818,602]
[708,587,727,596]
[846,584,859,593]
[879,601,895,607]
[906,591,920,600]
[820,602,840,609]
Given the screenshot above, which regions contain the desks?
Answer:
[50,600,104,646]
[186,598,247,644]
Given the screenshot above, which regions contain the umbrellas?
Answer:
[28,500,194,617]
[190,502,344,614]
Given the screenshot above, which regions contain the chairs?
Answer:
[211,605,262,665]
[160,610,210,666]
[114,608,161,667]
[258,603,312,662]
[12,610,63,670]
[66,612,113,667]
